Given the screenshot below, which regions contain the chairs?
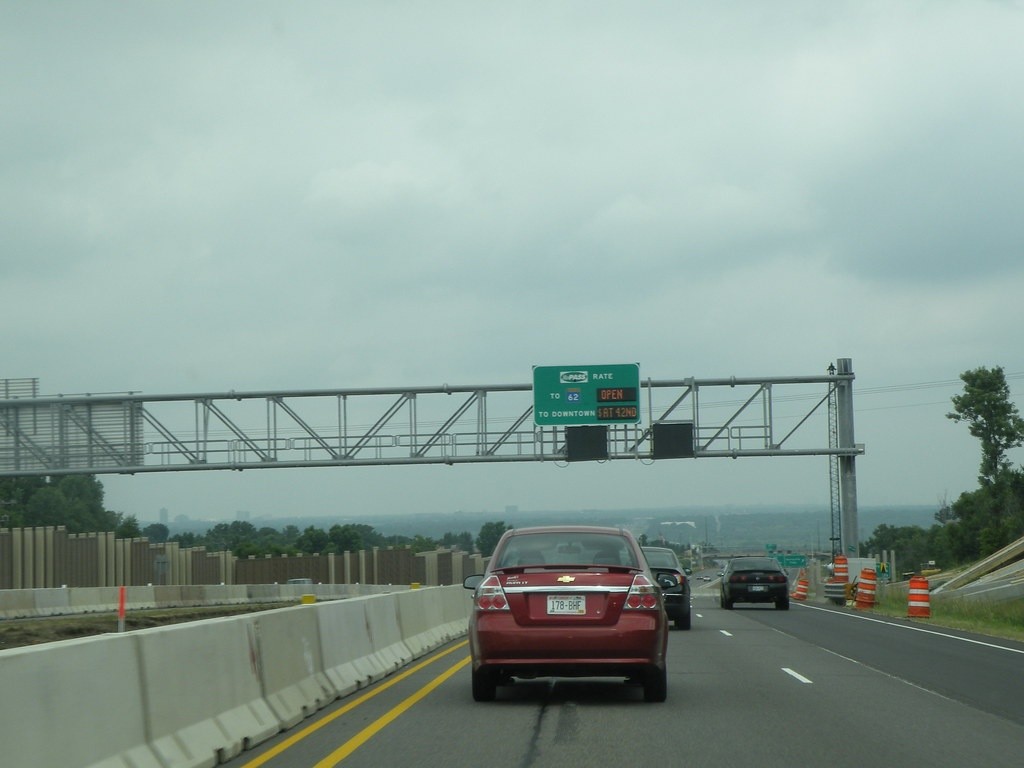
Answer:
[517,549,545,565]
[592,550,622,565]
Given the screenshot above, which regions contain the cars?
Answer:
[717,556,792,611]
[462,525,678,706]
[703,576,711,581]
[640,545,696,632]
[697,576,702,580]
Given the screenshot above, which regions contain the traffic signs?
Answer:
[532,363,643,426]
